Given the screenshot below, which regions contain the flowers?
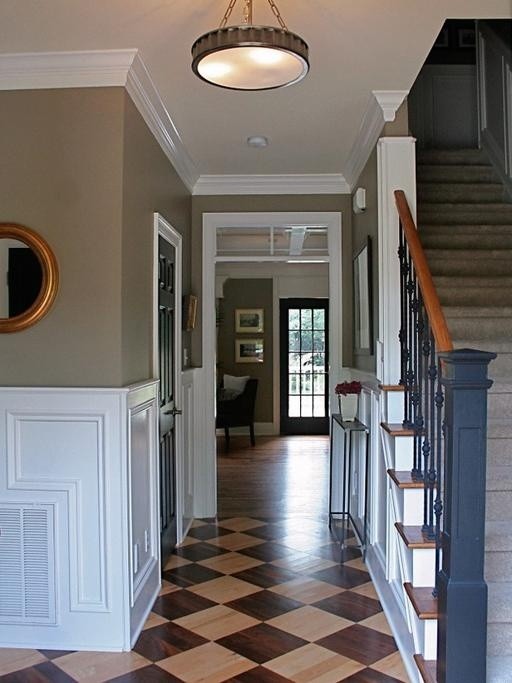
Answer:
[336,380,362,394]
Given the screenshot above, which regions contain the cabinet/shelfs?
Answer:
[329,413,370,564]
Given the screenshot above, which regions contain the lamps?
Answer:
[188,0,310,91]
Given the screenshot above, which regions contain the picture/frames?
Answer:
[353,234,374,357]
[232,307,266,364]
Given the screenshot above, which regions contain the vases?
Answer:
[339,394,358,423]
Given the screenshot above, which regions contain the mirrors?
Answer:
[0,222,59,333]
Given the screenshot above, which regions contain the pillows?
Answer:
[217,374,250,403]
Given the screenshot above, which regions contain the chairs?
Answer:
[216,372,258,450]
